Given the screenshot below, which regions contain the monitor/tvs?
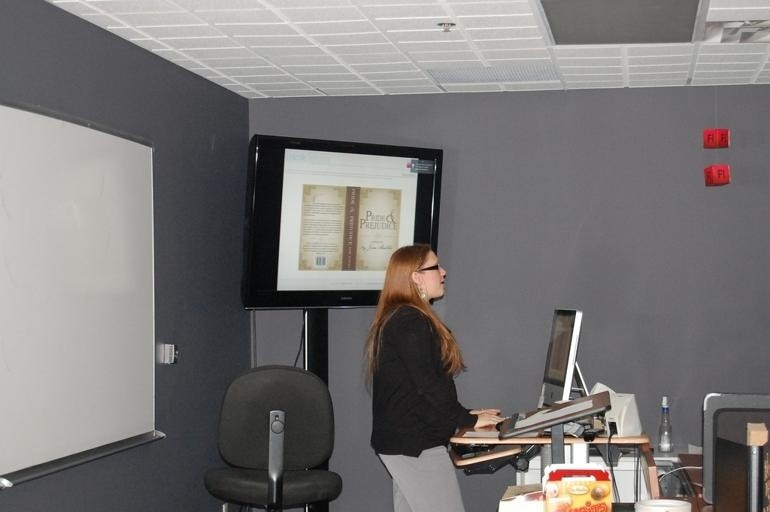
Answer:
[537,307,591,410]
[238,131,445,310]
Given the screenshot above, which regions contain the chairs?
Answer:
[204,364,342,511]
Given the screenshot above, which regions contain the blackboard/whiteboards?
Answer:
[0,100,168,489]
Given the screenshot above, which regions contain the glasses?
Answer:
[421,264,441,272]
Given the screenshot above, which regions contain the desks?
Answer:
[449,416,651,468]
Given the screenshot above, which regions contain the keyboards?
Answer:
[450,439,496,460]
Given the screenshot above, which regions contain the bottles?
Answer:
[658,396,672,451]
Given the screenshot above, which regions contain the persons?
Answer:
[360,243,504,512]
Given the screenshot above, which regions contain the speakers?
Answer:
[604,393,642,437]
